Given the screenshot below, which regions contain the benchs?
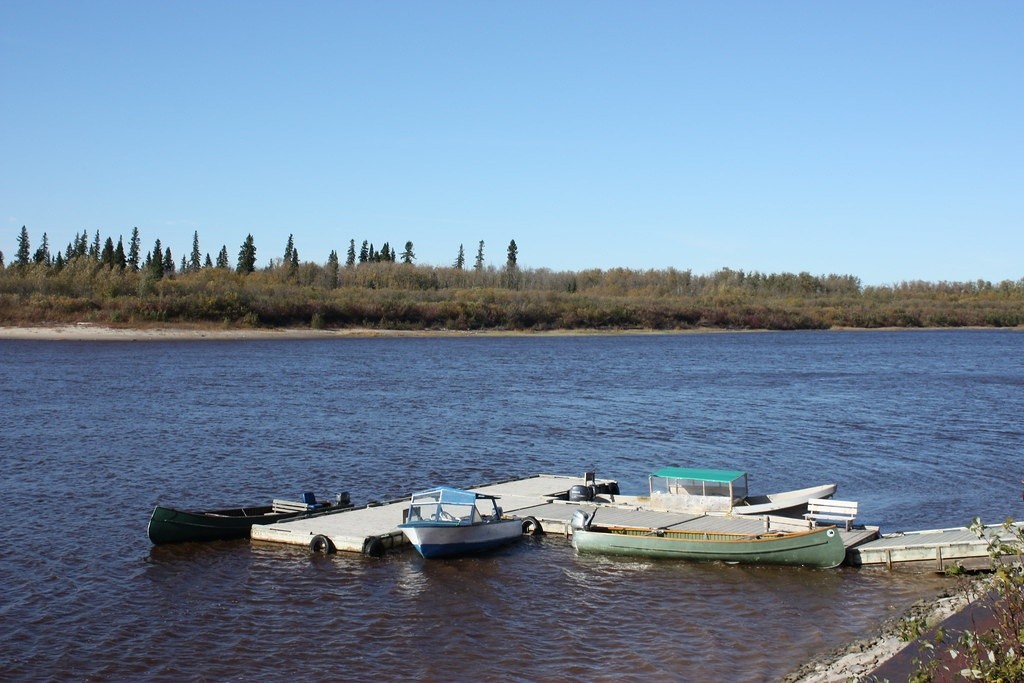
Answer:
[803,498,859,531]
[264,498,308,514]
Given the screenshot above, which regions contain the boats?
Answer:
[591,464,838,519]
[396,484,523,559]
[569,506,846,567]
[146,490,356,545]
